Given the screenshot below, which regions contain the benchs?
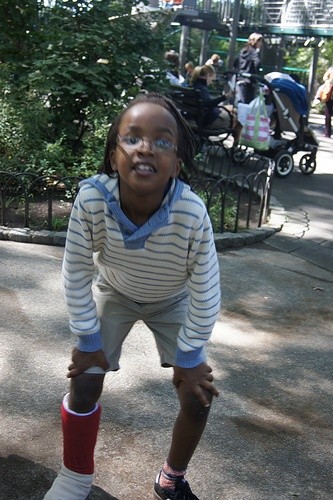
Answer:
[141,78,242,162]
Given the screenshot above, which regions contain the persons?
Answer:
[44,90,221,500]
[159,48,181,86]
[187,64,244,152]
[311,66,333,138]
[237,32,263,105]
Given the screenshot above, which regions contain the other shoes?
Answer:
[153,470,199,500]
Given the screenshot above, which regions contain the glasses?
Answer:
[118,134,179,154]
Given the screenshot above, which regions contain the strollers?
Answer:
[232,71,319,178]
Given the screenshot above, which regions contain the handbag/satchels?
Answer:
[317,81,333,102]
[239,93,271,150]
